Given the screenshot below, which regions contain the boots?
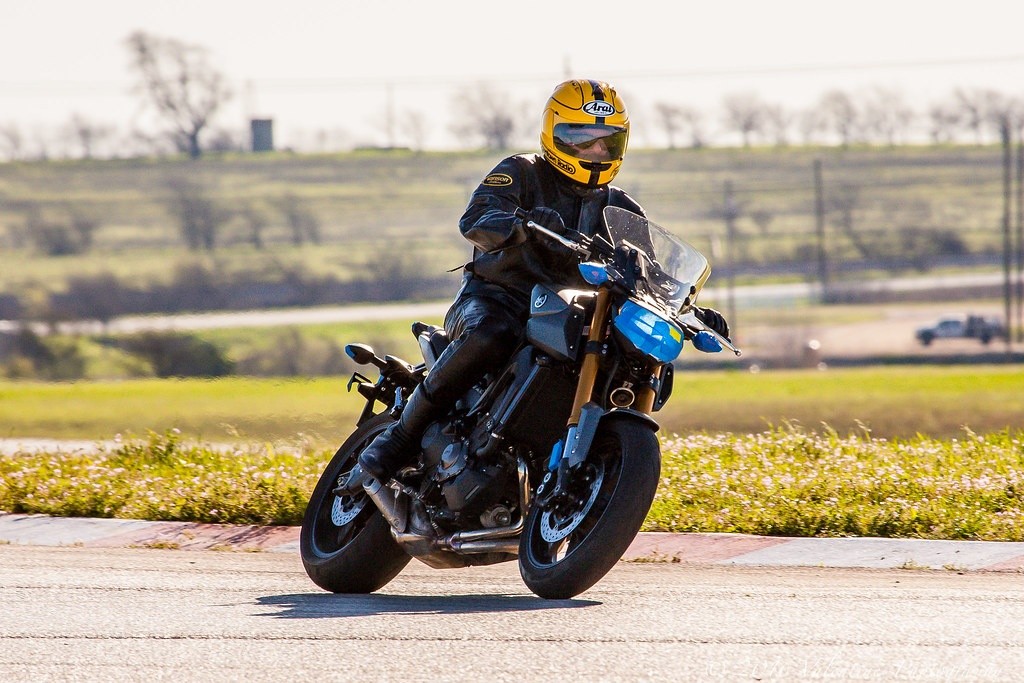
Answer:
[357,381,439,483]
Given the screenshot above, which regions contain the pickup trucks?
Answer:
[917,315,1000,344]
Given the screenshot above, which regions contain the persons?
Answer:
[358,80,731,479]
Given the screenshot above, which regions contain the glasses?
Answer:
[562,132,620,153]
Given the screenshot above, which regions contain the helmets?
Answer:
[540,79,630,189]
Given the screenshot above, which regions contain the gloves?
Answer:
[696,307,730,339]
[522,207,565,240]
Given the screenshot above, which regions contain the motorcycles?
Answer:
[299,210,742,599]
